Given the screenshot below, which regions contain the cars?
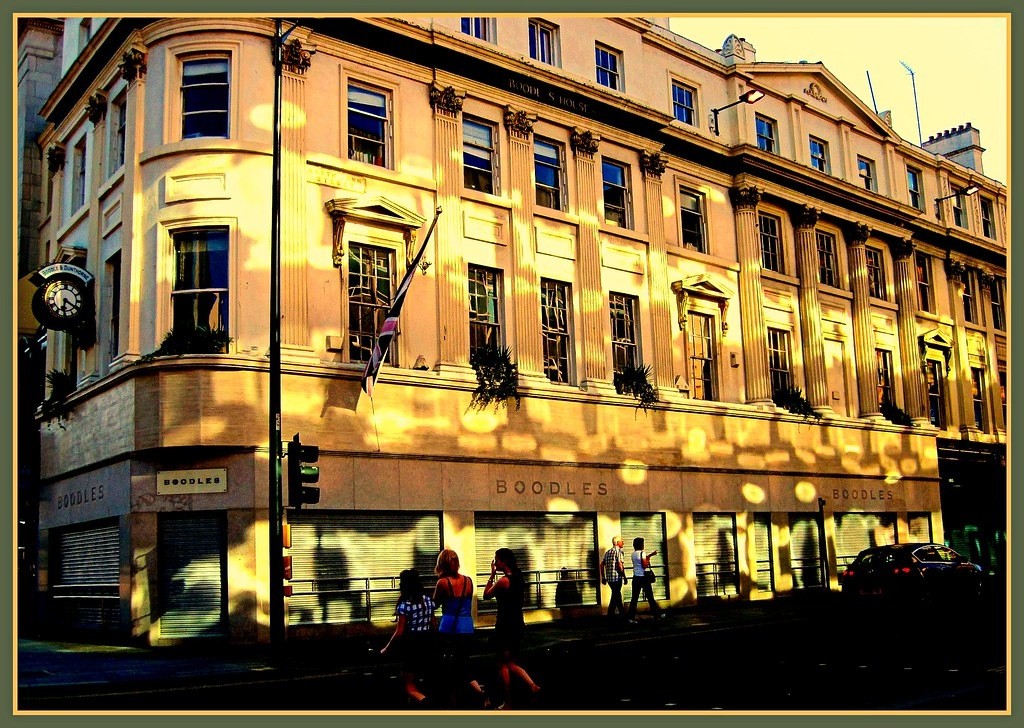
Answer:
[840,542,984,599]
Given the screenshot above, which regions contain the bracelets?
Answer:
[602,577,604,578]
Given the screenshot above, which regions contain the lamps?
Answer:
[934,185,980,219]
[711,90,765,136]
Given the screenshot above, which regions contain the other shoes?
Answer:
[628,618,639,624]
[657,611,667,621]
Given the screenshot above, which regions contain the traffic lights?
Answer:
[288,442,320,509]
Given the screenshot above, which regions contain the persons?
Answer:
[627,537,666,623]
[483,548,543,710]
[413,355,429,371]
[430,550,506,711]
[601,536,628,620]
[379,569,436,709]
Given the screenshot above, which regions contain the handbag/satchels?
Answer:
[438,635,479,678]
[644,571,655,583]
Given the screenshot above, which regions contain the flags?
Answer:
[363,231,428,398]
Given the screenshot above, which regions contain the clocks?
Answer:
[29,262,96,338]
